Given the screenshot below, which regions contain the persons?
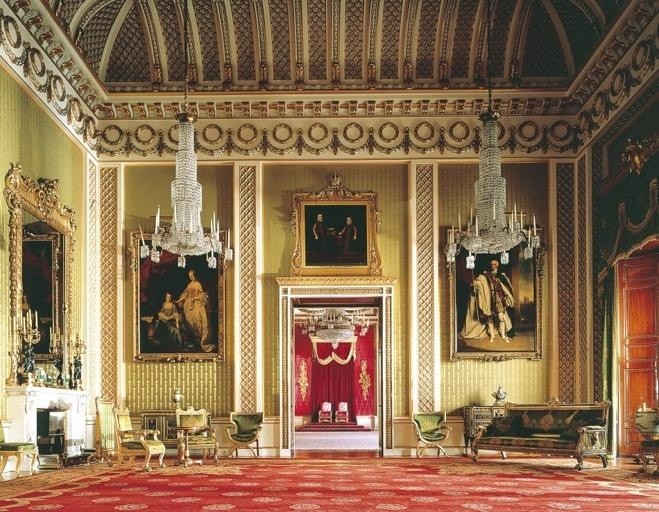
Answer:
[338,213,358,263]
[173,269,218,353]
[312,210,331,263]
[146,290,181,353]
[457,258,517,344]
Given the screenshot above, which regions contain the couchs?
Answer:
[470,399,613,471]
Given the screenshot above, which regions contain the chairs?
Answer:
[113,404,160,471]
[318,401,333,423]
[334,401,348,423]
[225,410,265,461]
[174,404,219,467]
[410,410,455,459]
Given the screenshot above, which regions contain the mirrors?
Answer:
[3,163,79,367]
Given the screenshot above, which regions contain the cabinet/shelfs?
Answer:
[463,404,506,455]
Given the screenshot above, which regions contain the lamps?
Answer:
[46,321,66,386]
[298,306,371,342]
[438,1,543,271]
[10,300,45,385]
[66,326,87,390]
[132,1,237,268]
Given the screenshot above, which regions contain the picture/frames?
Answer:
[132,227,229,365]
[289,168,383,274]
[446,226,543,361]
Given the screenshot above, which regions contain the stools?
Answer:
[0,440,41,482]
[637,438,658,475]
